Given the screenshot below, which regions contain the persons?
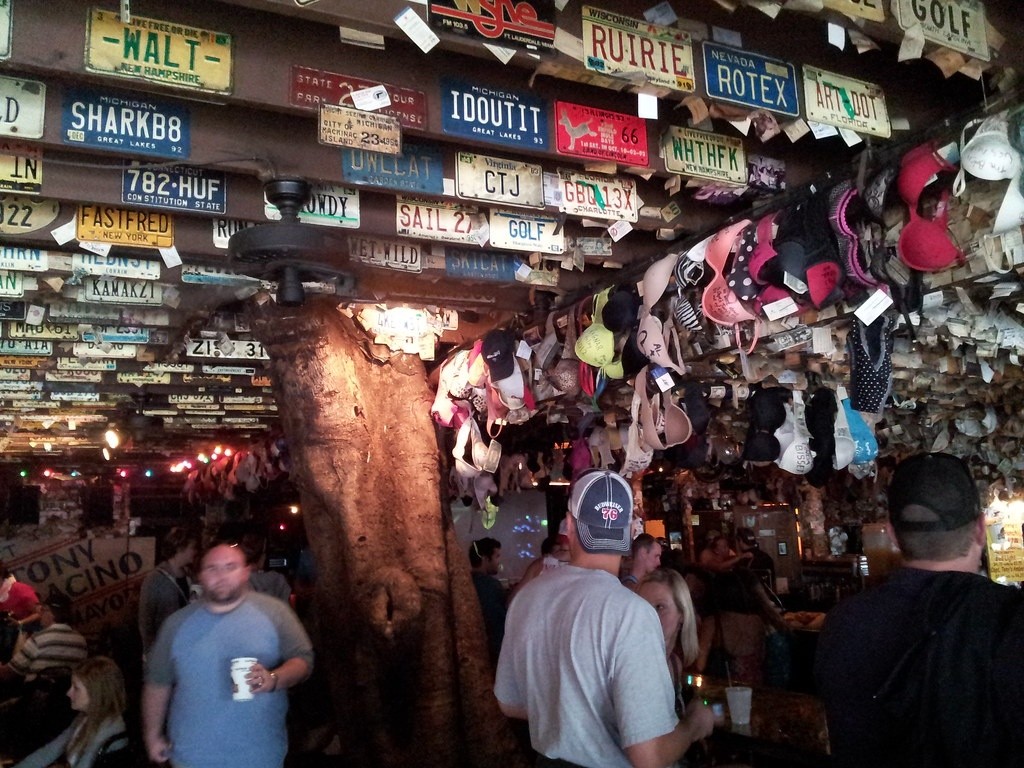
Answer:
[811,452,1024,767]
[0,559,139,768]
[494,468,714,768]
[142,539,314,768]
[466,518,571,674]
[245,539,298,611]
[139,525,198,652]
[623,528,777,768]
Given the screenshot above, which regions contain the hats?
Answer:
[558,517,568,535]
[41,591,73,608]
[888,451,982,532]
[737,526,757,546]
[567,468,633,555]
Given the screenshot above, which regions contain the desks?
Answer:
[682,672,831,768]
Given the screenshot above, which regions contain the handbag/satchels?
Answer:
[0,610,17,662]
[703,615,733,679]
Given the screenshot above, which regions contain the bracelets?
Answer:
[269,671,280,692]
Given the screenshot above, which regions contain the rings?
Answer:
[258,676,264,685]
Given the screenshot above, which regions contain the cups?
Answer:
[229,657,258,702]
[725,686,752,725]
